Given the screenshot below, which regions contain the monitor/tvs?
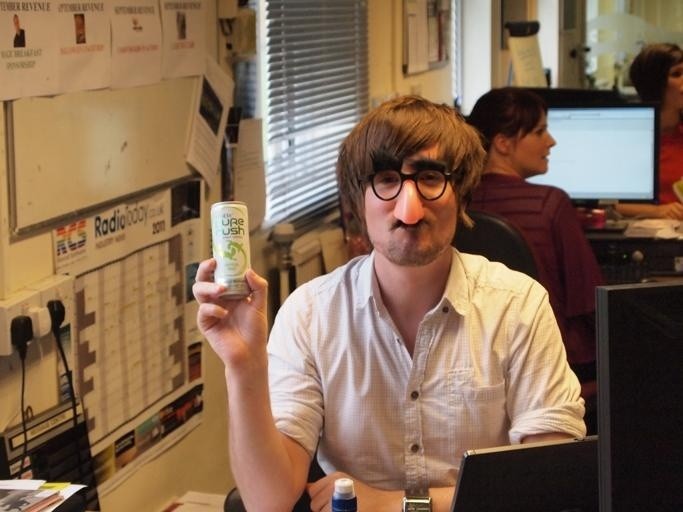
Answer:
[592,282,683,512]
[524,104,659,232]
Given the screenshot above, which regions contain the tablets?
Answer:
[449,434,598,512]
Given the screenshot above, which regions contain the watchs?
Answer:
[402,488,432,512]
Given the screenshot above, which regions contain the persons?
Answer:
[616,42,683,219]
[13,14,25,47]
[193,94,587,512]
[464,88,609,413]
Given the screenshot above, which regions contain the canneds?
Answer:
[208,201,252,300]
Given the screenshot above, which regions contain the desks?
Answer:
[583,217,683,286]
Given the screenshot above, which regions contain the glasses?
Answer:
[371,169,455,201]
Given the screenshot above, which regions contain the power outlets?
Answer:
[31,274,74,331]
[1,289,39,358]
